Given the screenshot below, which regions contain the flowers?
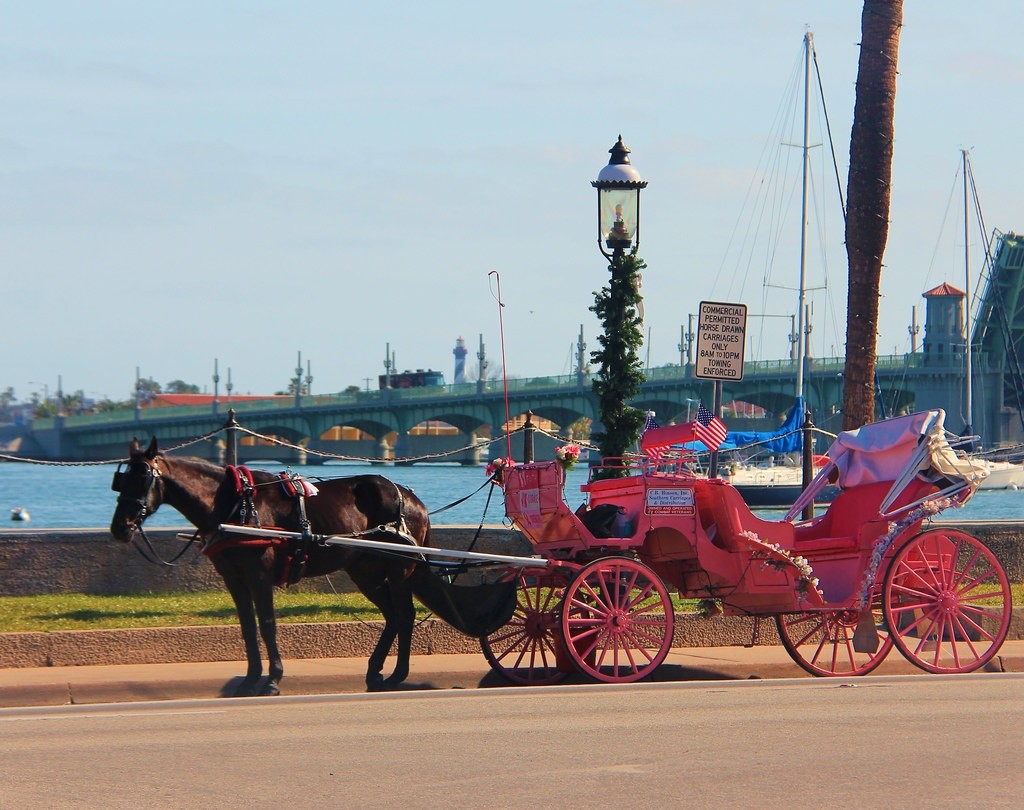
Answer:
[485,456,514,482]
[737,495,956,607]
[555,444,580,484]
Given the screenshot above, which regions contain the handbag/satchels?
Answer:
[575,503,628,539]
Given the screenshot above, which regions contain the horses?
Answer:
[112,432,440,696]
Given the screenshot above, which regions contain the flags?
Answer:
[690,404,728,452]
[641,414,672,465]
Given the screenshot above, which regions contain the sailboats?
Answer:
[636,32,990,511]
[875,149,1024,490]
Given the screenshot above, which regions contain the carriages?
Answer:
[111,270,1012,697]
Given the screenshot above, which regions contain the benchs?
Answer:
[580,419,700,500]
[787,477,934,563]
[696,479,794,551]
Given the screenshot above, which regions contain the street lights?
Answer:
[590,134,648,612]
